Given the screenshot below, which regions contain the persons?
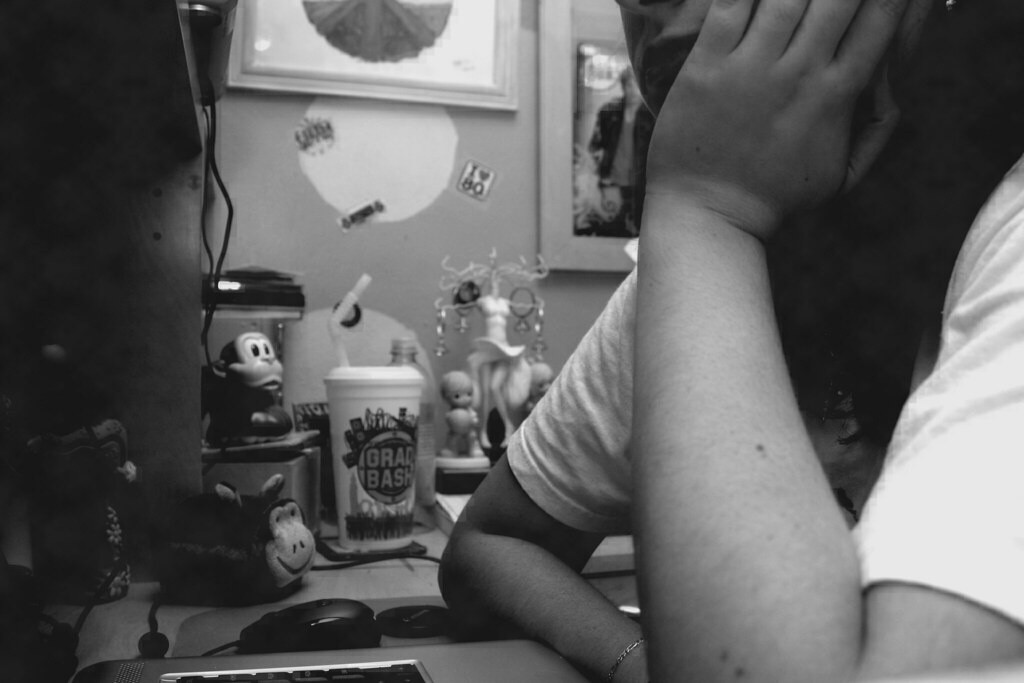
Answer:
[519,359,554,416]
[436,0,1023,683]
[586,65,655,237]
[436,370,486,459]
[464,278,528,449]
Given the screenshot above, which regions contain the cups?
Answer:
[323,366,424,553]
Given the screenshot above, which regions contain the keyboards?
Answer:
[161,659,435,683]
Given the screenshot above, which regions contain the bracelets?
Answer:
[606,636,647,683]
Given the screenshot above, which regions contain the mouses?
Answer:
[238,597,382,653]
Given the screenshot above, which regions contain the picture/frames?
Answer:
[538,0,664,274]
[224,0,522,111]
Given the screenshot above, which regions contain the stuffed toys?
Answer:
[150,471,316,611]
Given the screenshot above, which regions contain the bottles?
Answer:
[383,337,436,533]
